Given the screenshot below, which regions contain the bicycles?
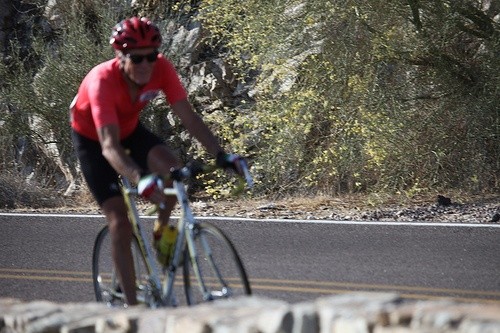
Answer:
[91,162,252,309]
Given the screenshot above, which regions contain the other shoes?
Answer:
[152,238,168,266]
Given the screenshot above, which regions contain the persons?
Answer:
[69,17,245,309]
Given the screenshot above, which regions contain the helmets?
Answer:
[109,15,163,51]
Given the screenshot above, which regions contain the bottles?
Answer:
[158,225,178,266]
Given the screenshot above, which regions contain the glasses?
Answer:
[121,51,160,64]
